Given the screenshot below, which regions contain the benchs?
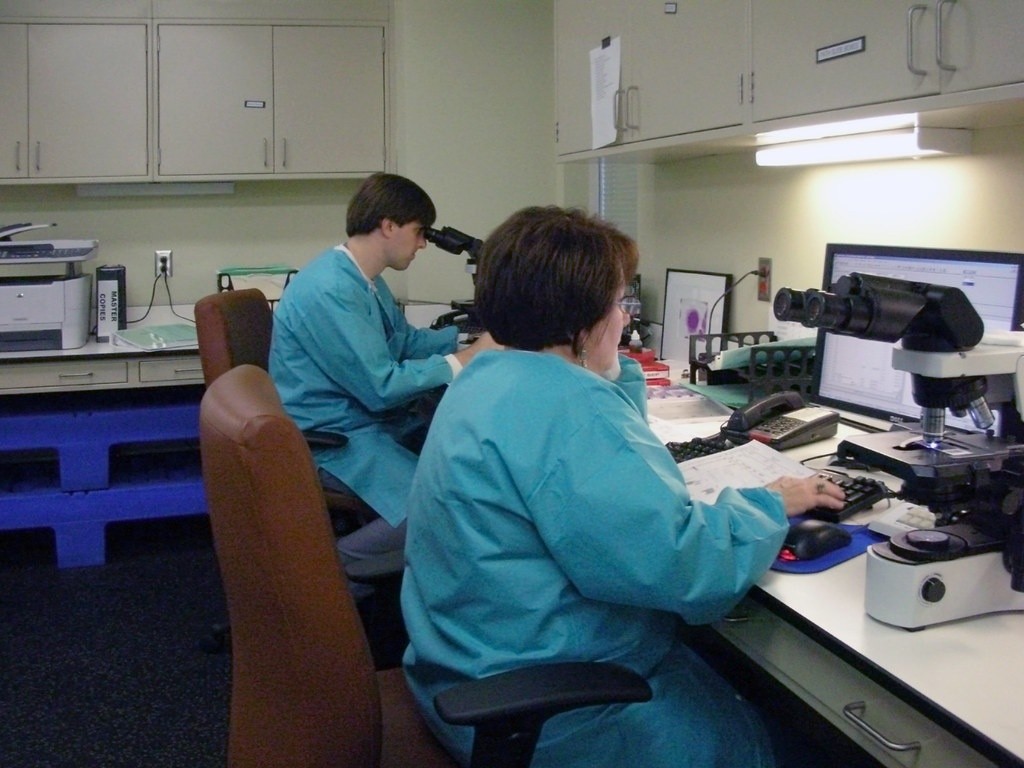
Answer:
[0,384,208,570]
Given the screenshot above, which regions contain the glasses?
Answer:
[608,296,642,315]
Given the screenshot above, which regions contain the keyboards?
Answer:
[665,441,886,523]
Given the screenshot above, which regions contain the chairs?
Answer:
[197,363,651,768]
[194,287,385,537]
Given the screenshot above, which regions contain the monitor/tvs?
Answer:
[813,243,1024,442]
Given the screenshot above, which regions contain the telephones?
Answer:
[720,391,839,452]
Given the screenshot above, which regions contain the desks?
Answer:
[644,360,1024,768]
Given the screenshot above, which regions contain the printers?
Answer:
[0,224,99,353]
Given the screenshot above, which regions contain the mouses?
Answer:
[776,519,852,561]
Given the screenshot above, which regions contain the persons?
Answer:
[400,204,846,768]
[268,172,506,611]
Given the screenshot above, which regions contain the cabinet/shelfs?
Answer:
[552,0,1024,167]
[0,0,397,186]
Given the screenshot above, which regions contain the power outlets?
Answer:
[154,250,173,278]
[757,257,773,302]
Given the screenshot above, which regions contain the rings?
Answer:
[818,484,825,492]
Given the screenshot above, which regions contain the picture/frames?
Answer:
[660,268,733,362]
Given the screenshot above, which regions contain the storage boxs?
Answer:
[214,266,300,313]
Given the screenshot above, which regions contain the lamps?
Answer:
[74,182,236,199]
[753,126,973,169]
